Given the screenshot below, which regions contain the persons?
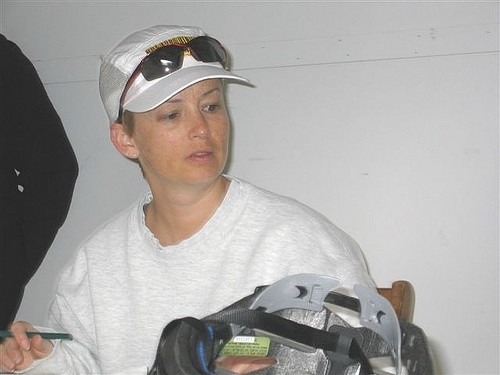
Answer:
[0,35,79,344]
[0,25,375,375]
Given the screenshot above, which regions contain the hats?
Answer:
[99,24,251,131]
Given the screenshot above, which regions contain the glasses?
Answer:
[118,36,228,123]
